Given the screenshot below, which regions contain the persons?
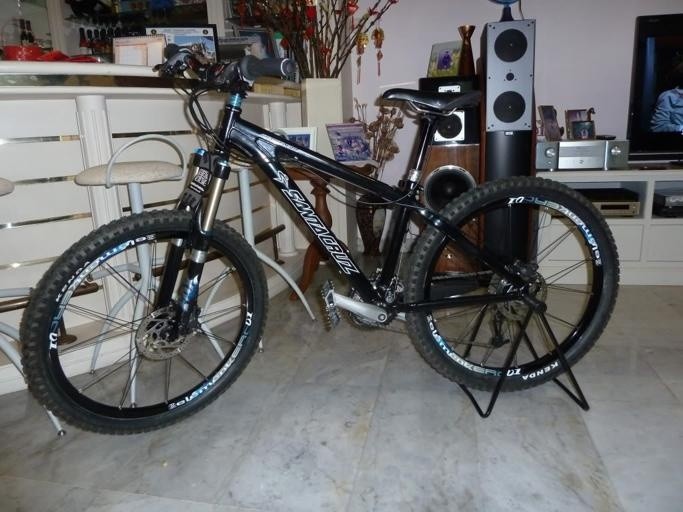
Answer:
[333,131,368,160]
[441,50,452,72]
[648,51,683,132]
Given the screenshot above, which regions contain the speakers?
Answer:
[419,76,481,144]
[420,144,480,272]
[481,17,537,265]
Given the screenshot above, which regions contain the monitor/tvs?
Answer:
[626,14,683,169]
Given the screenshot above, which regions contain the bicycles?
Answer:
[20,44,620,436]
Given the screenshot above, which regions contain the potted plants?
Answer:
[353,98,406,255]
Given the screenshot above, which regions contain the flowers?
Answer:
[229,0,401,79]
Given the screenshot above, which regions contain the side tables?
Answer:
[282,164,374,302]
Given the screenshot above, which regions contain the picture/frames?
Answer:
[265,125,318,153]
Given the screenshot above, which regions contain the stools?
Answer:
[74,132,224,412]
[194,153,318,356]
[0,178,67,437]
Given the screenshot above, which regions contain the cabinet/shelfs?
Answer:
[44,0,354,79]
[535,166,682,285]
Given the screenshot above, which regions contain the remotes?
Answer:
[595,135,615,140]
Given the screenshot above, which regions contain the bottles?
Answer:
[19,19,34,45]
[77,26,129,62]
